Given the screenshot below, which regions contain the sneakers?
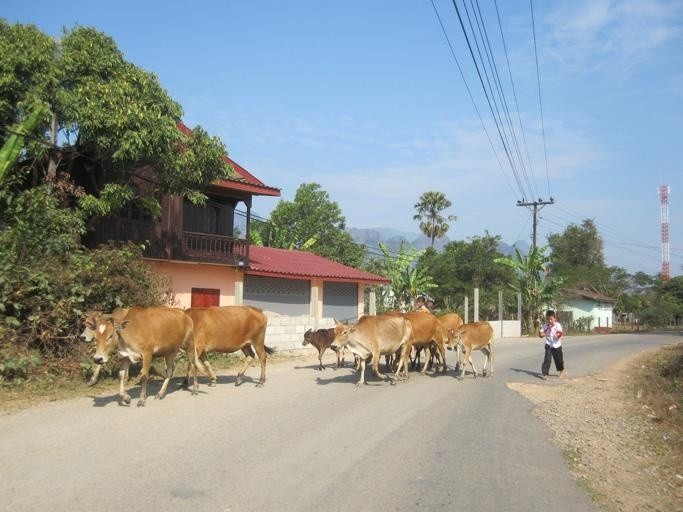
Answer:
[542,369,567,380]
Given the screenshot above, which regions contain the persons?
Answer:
[416,296,428,311]
[537,310,564,380]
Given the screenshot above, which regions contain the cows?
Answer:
[302,327,345,371]
[446,321,494,381]
[331,309,463,384]
[79,308,209,408]
[180,305,277,389]
